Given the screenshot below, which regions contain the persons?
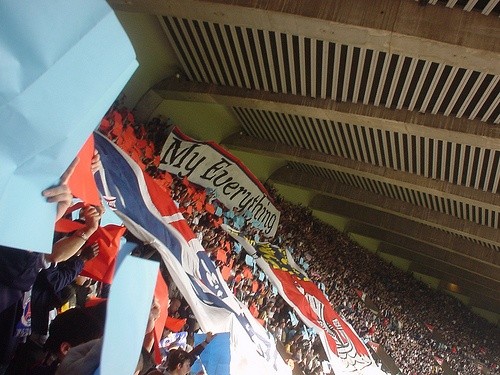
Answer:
[0,90,499,375]
